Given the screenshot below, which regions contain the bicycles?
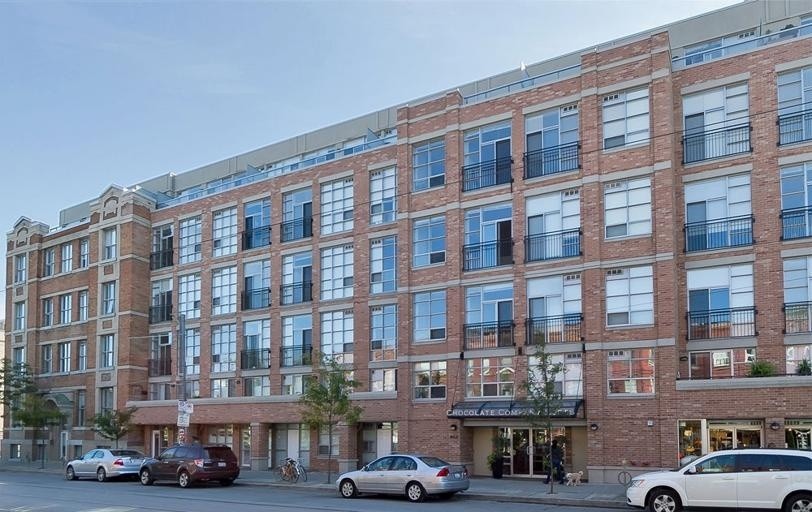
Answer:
[273,457,310,483]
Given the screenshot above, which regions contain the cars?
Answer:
[721,437,734,448]
[334,453,471,503]
[64,449,147,481]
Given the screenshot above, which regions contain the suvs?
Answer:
[141,443,240,488]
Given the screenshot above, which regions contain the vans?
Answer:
[623,446,812,512]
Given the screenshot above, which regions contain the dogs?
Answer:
[566,470,584,486]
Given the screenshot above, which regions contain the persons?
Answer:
[543,440,564,484]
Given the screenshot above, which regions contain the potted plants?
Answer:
[486,449,504,479]
